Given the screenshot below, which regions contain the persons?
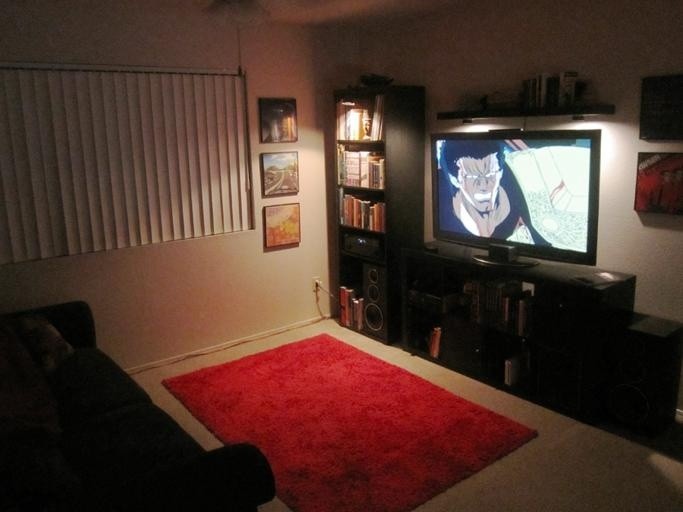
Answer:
[647,169,682,213]
[433,138,519,241]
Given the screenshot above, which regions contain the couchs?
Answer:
[0,299,277,511]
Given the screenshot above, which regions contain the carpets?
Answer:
[161,333,539,512]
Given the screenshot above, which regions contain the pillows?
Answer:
[17,316,78,372]
[0,322,33,367]
[1,368,62,434]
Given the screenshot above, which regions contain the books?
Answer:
[504,289,532,338]
[336,94,385,232]
[340,285,363,332]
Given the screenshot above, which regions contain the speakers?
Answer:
[607,316,683,438]
[363,263,401,345]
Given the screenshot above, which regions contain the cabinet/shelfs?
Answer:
[399,238,636,428]
[326,85,426,333]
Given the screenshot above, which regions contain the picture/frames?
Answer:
[261,152,299,196]
[259,97,298,143]
[264,203,300,248]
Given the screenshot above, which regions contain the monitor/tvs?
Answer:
[431,130,601,270]
